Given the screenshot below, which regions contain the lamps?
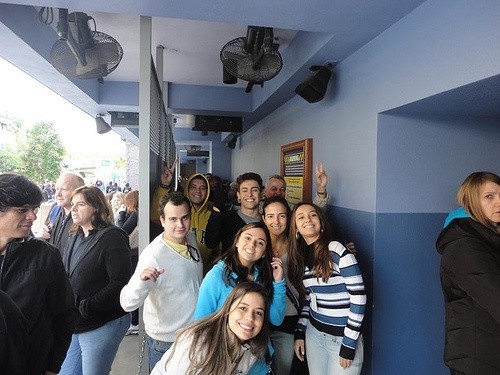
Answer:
[95,117,112,134]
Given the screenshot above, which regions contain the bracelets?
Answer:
[160,185,168,189]
[317,191,326,195]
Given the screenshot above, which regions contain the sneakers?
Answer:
[125,322,140,336]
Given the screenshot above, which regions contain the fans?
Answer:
[220,26,284,82]
[50,12,123,81]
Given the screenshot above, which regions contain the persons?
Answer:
[0,157,365,375]
[435,171,500,375]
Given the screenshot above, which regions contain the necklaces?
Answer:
[273,239,286,257]
